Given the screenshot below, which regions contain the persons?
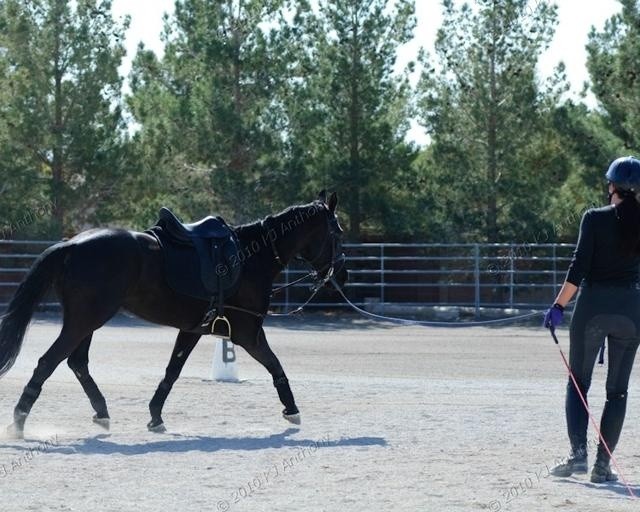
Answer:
[544,156,640,483]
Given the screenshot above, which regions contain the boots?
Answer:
[549,444,588,478]
[590,456,618,483]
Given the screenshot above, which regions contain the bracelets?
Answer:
[553,303,565,312]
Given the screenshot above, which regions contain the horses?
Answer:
[0,190,349,440]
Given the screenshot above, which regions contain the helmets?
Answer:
[605,155,640,193]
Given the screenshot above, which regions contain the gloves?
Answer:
[543,303,565,329]
